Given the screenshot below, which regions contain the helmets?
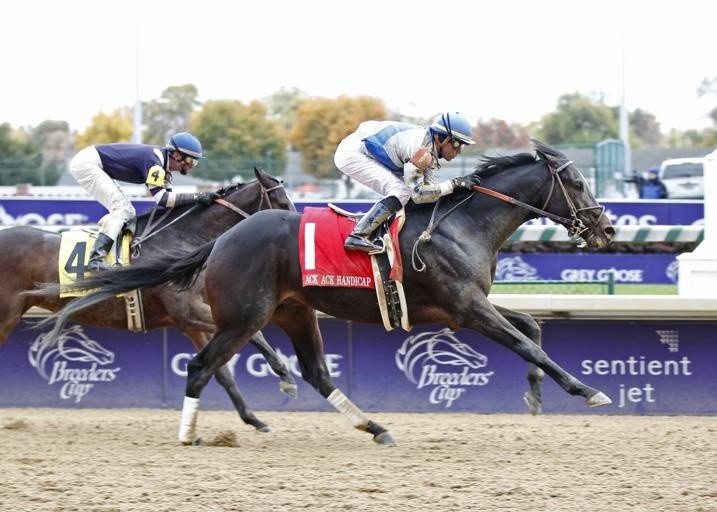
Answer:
[430,111,476,145]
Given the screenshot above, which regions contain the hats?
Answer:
[648,167,660,175]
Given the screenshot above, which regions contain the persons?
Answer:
[69,132,221,271]
[334,111,482,253]
[639,166,668,199]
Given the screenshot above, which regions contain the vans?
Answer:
[657,155,704,201]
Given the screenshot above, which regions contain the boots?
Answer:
[344,202,391,251]
[88,232,116,272]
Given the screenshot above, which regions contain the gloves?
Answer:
[452,174,481,190]
[198,191,221,206]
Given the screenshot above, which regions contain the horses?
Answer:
[0,166,298,434]
[10,139,615,447]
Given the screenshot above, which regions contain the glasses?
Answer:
[185,157,199,167]
[451,139,464,154]
